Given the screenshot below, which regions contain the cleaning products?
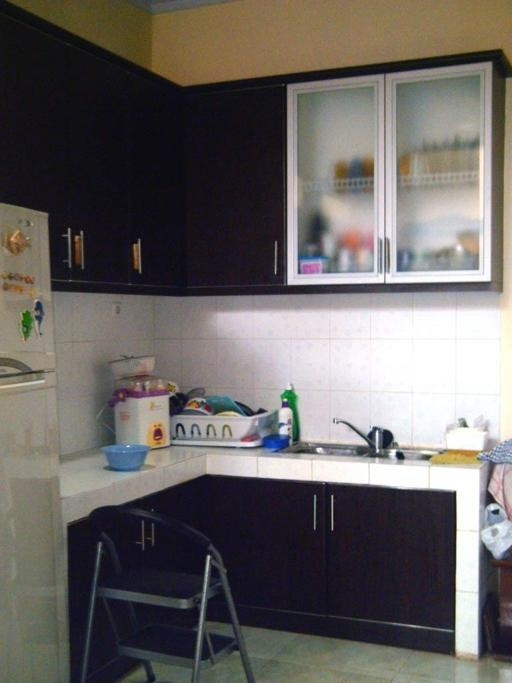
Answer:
[277,383,300,445]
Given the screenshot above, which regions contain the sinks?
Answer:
[362,447,443,461]
[276,441,370,456]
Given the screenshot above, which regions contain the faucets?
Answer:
[333,417,385,456]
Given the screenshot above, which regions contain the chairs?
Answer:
[80,505,256,681]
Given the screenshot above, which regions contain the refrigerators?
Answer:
[1,201,71,683]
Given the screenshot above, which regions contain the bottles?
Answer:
[277,382,300,446]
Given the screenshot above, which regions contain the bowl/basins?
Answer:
[108,355,157,380]
[180,396,214,416]
[101,443,151,473]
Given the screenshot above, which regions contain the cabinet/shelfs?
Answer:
[67,475,205,681]
[1,1,511,297]
[206,476,455,653]
